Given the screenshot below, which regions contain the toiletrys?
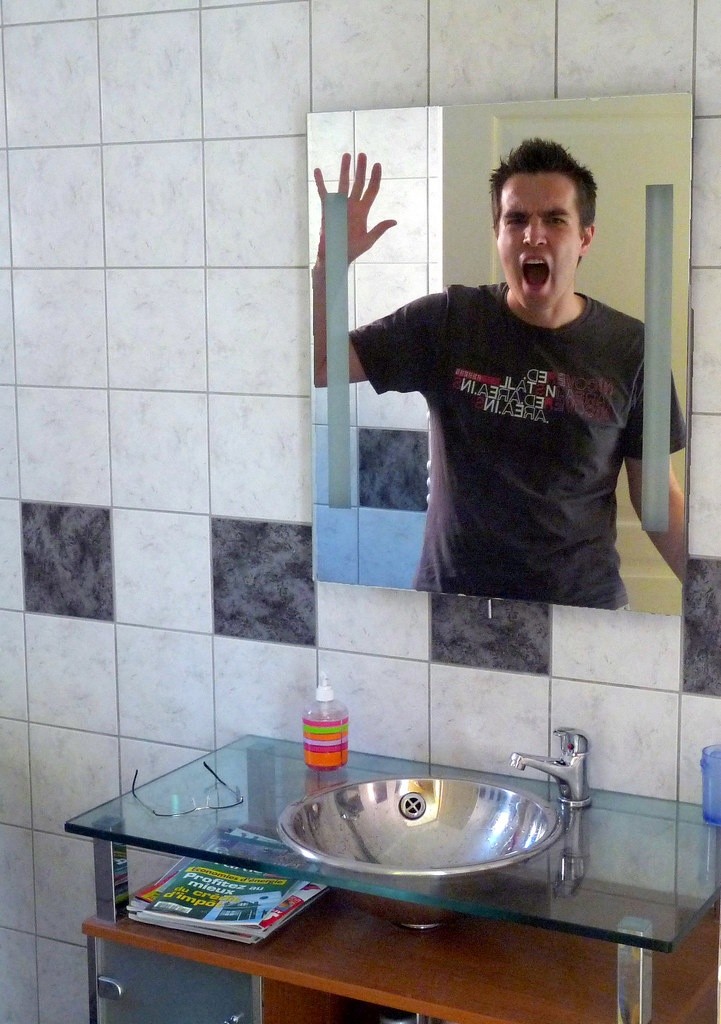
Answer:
[300,665,350,771]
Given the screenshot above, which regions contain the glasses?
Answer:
[131,761,243,817]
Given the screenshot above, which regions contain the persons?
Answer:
[313,141,686,610]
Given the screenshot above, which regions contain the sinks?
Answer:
[277,773,565,931]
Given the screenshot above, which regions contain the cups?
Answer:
[700,744,721,826]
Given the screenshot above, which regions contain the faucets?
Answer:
[551,807,589,901]
[508,726,593,809]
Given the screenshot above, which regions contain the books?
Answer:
[125,822,331,944]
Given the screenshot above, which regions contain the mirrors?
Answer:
[305,91,695,616]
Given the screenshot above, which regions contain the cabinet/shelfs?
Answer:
[64,734,717,1024]
